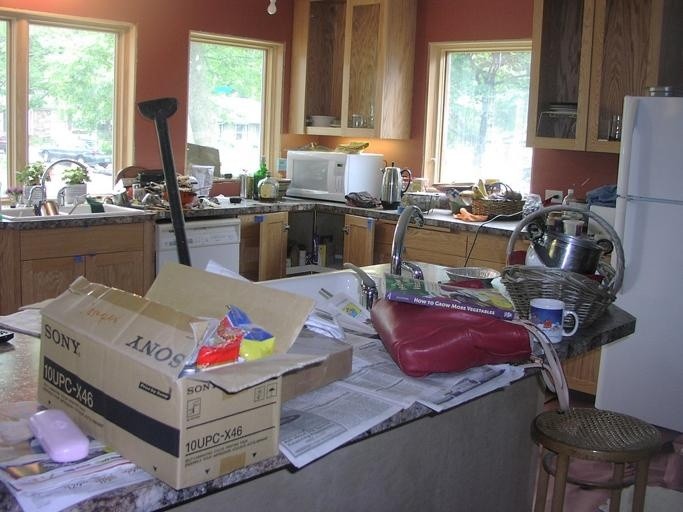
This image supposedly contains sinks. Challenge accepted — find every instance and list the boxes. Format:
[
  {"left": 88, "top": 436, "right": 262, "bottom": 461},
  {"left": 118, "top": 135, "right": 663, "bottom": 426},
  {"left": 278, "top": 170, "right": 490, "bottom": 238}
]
[
  {"left": 355, "top": 259, "right": 451, "bottom": 292},
  {"left": 0, "top": 206, "right": 68, "bottom": 221},
  {"left": 253, "top": 269, "right": 386, "bottom": 309},
  {"left": 55, "top": 203, "right": 144, "bottom": 216}
]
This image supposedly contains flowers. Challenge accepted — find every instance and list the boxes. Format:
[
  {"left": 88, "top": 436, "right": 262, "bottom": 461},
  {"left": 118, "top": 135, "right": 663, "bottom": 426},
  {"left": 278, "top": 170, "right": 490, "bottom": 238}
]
[{"left": 7, "top": 187, "right": 22, "bottom": 198}]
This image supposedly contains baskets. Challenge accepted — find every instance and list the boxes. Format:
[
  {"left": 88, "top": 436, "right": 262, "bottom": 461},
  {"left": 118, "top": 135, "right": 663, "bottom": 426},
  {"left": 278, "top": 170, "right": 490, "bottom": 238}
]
[
  {"left": 470, "top": 198, "right": 524, "bottom": 221},
  {"left": 502, "top": 202, "right": 625, "bottom": 330}
]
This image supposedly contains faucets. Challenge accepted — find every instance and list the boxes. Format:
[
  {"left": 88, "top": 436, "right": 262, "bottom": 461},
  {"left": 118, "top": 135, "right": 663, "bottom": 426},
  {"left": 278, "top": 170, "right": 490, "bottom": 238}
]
[
  {"left": 391, "top": 204, "right": 425, "bottom": 276},
  {"left": 38, "top": 158, "right": 89, "bottom": 206}
]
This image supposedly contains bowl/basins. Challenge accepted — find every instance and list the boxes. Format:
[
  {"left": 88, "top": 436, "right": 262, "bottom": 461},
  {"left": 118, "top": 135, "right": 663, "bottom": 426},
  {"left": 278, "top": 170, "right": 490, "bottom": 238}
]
[{"left": 645, "top": 84, "right": 682, "bottom": 96}]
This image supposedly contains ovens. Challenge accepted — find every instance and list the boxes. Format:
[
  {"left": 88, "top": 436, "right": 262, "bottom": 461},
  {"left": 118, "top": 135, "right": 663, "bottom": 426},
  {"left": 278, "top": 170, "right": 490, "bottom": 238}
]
[{"left": 156, "top": 215, "right": 240, "bottom": 282}]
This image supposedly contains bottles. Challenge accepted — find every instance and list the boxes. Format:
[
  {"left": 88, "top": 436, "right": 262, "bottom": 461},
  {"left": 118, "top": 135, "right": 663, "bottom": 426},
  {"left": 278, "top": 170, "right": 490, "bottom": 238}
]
[
  {"left": 298, "top": 246, "right": 304, "bottom": 266},
  {"left": 317, "top": 286, "right": 370, "bottom": 323},
  {"left": 562, "top": 187, "right": 577, "bottom": 218},
  {"left": 240, "top": 156, "right": 279, "bottom": 202},
  {"left": 130, "top": 183, "right": 141, "bottom": 199},
  {"left": 317, "top": 238, "right": 326, "bottom": 267}
]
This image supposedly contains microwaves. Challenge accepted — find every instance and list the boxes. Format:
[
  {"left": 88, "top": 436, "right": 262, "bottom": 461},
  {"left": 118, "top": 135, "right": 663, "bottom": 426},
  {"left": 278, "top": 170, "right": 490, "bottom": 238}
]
[{"left": 285, "top": 149, "right": 383, "bottom": 204}]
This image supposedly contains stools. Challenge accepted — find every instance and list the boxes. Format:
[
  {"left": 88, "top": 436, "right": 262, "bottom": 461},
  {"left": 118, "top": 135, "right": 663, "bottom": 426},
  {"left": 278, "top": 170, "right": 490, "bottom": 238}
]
[{"left": 531, "top": 407, "right": 661, "bottom": 512}]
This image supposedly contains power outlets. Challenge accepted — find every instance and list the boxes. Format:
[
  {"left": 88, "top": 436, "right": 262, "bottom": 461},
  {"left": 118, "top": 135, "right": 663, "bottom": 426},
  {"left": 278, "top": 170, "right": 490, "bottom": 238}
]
[{"left": 545, "top": 190, "right": 563, "bottom": 204}]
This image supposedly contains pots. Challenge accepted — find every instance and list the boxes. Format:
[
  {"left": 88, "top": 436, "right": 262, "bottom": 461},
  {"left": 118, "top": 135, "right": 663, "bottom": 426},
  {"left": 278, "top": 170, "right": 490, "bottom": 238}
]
[{"left": 524, "top": 220, "right": 613, "bottom": 276}]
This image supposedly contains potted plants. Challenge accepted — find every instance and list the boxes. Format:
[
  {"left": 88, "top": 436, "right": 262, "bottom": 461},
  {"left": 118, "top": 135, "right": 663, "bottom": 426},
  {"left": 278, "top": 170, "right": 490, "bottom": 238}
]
[
  {"left": 61, "top": 167, "right": 90, "bottom": 204},
  {"left": 16, "top": 161, "right": 50, "bottom": 202}
]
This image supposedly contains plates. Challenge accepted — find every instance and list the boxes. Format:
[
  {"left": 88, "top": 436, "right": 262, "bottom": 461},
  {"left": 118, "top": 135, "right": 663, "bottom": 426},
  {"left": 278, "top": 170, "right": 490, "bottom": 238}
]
[
  {"left": 305, "top": 115, "right": 340, "bottom": 127},
  {"left": 444, "top": 266, "right": 501, "bottom": 283}
]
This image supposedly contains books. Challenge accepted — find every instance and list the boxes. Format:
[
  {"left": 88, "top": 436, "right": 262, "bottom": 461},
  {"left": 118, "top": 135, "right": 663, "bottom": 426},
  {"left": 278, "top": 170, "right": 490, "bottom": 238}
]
[{"left": 379, "top": 272, "right": 514, "bottom": 322}]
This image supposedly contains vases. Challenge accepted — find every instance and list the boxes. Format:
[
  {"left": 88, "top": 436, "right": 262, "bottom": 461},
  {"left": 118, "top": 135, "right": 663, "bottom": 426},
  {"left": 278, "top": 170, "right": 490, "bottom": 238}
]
[{"left": 10, "top": 195, "right": 18, "bottom": 208}]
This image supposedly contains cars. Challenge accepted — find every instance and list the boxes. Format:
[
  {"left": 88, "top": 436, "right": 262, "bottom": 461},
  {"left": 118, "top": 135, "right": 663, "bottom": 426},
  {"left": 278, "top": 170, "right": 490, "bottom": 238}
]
[{"left": 0, "top": 127, "right": 112, "bottom": 171}]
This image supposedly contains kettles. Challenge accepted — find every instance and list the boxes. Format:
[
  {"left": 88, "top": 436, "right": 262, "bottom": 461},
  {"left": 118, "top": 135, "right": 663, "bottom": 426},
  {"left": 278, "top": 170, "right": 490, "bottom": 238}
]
[{"left": 378, "top": 162, "right": 410, "bottom": 209}]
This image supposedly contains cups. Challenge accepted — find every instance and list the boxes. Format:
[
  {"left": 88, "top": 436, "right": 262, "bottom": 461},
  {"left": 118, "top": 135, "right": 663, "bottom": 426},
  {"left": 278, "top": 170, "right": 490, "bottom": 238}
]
[
  {"left": 191, "top": 164, "right": 215, "bottom": 198},
  {"left": 562, "top": 219, "right": 583, "bottom": 236},
  {"left": 348, "top": 99, "right": 374, "bottom": 128},
  {"left": 527, "top": 297, "right": 578, "bottom": 345}
]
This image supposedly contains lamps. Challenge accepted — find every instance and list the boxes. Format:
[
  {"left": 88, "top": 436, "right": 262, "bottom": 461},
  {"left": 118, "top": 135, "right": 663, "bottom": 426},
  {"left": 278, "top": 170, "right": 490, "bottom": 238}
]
[{"left": 267, "top": 0, "right": 277, "bottom": 15}]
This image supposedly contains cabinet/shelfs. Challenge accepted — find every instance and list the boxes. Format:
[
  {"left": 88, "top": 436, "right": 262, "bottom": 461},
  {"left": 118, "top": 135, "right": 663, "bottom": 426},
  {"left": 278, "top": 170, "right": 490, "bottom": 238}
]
[
  {"left": 526, "top": 0, "right": 665, "bottom": 154},
  {"left": 1, "top": 222, "right": 156, "bottom": 317},
  {"left": 289, "top": 0, "right": 417, "bottom": 139}
]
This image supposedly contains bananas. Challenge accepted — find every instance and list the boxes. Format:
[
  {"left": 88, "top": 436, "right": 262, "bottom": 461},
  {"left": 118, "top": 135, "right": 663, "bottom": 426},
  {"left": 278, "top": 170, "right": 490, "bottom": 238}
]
[{"left": 459, "top": 179, "right": 488, "bottom": 199}]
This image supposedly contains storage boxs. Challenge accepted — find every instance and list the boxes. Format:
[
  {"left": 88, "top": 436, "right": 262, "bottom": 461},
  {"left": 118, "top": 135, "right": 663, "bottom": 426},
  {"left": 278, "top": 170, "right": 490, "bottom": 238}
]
[{"left": 38, "top": 260, "right": 327, "bottom": 491}]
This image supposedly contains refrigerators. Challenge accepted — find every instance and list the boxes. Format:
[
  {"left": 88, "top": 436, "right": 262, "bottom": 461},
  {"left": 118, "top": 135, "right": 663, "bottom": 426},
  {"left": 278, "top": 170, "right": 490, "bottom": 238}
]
[{"left": 591, "top": 94, "right": 682, "bottom": 434}]
